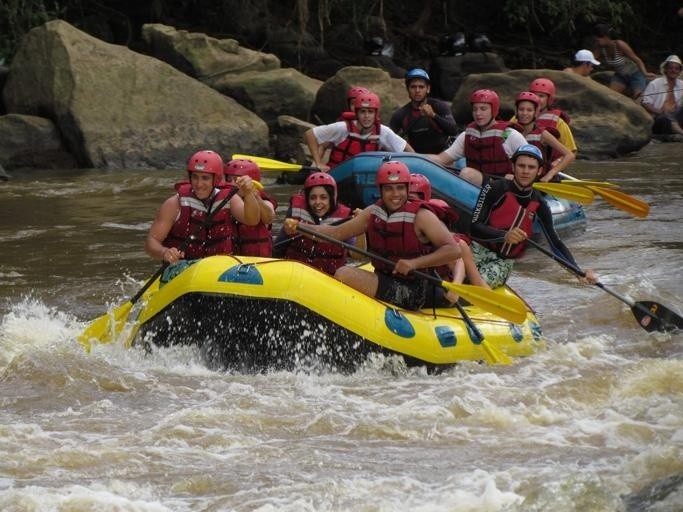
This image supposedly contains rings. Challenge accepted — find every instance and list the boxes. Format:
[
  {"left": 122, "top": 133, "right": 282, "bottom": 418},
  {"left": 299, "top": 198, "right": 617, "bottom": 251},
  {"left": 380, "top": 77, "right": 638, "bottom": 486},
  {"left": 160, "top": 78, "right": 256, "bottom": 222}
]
[
  {"left": 168, "top": 255, "right": 173, "bottom": 259},
  {"left": 174, "top": 251, "right": 179, "bottom": 257}
]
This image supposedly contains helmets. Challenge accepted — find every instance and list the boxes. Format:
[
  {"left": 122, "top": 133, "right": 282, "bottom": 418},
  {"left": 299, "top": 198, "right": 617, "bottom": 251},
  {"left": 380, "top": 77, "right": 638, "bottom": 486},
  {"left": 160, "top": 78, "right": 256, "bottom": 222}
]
[
  {"left": 469, "top": 89, "right": 500, "bottom": 118},
  {"left": 516, "top": 91, "right": 540, "bottom": 118},
  {"left": 303, "top": 172, "right": 338, "bottom": 205},
  {"left": 409, "top": 173, "right": 432, "bottom": 201},
  {"left": 530, "top": 78, "right": 556, "bottom": 106},
  {"left": 187, "top": 150, "right": 225, "bottom": 186},
  {"left": 376, "top": 160, "right": 409, "bottom": 193},
  {"left": 660, "top": 54, "right": 683, "bottom": 78},
  {"left": 511, "top": 144, "right": 543, "bottom": 164},
  {"left": 346, "top": 86, "right": 368, "bottom": 101},
  {"left": 354, "top": 92, "right": 380, "bottom": 121},
  {"left": 404, "top": 67, "right": 432, "bottom": 85},
  {"left": 223, "top": 159, "right": 261, "bottom": 184}
]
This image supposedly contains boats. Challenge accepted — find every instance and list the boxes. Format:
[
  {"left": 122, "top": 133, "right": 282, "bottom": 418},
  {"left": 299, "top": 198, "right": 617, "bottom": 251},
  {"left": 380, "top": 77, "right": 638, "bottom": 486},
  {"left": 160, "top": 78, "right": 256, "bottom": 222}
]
[
  {"left": 125, "top": 253, "right": 544, "bottom": 379},
  {"left": 323, "top": 151, "right": 590, "bottom": 244}
]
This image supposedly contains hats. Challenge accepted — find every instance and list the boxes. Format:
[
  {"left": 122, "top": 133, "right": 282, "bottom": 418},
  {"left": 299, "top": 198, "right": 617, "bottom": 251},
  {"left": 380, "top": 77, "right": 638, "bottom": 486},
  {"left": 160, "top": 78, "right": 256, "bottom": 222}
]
[{"left": 576, "top": 49, "right": 602, "bottom": 67}]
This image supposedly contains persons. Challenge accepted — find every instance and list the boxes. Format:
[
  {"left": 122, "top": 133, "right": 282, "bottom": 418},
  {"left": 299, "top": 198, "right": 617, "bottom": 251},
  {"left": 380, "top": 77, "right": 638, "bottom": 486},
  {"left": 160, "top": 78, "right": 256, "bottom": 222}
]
[
  {"left": 389, "top": 67, "right": 459, "bottom": 155},
  {"left": 421, "top": 86, "right": 530, "bottom": 189},
  {"left": 641, "top": 54, "right": 683, "bottom": 135},
  {"left": 461, "top": 143, "right": 598, "bottom": 290},
  {"left": 271, "top": 171, "right": 367, "bottom": 282},
  {"left": 222, "top": 159, "right": 279, "bottom": 259},
  {"left": 303, "top": 90, "right": 417, "bottom": 175},
  {"left": 408, "top": 172, "right": 470, "bottom": 315},
  {"left": 466, "top": 28, "right": 491, "bottom": 53},
  {"left": 586, "top": 24, "right": 657, "bottom": 99},
  {"left": 311, "top": 85, "right": 369, "bottom": 168},
  {"left": 144, "top": 147, "right": 262, "bottom": 292},
  {"left": 561, "top": 48, "right": 601, "bottom": 80},
  {"left": 509, "top": 77, "right": 578, "bottom": 171},
  {"left": 361, "top": 26, "right": 399, "bottom": 57},
  {"left": 281, "top": 159, "right": 461, "bottom": 309},
  {"left": 510, "top": 90, "right": 577, "bottom": 197},
  {"left": 436, "top": 24, "right": 466, "bottom": 57}
]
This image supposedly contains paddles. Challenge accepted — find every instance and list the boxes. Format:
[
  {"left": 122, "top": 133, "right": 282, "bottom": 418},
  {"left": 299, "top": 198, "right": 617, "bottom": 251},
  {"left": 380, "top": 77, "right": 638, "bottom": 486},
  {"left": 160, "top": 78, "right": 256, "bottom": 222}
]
[
  {"left": 284, "top": 219, "right": 527, "bottom": 369},
  {"left": 78, "top": 179, "right": 263, "bottom": 354},
  {"left": 232, "top": 153, "right": 322, "bottom": 172},
  {"left": 444, "top": 167, "right": 650, "bottom": 218},
  {"left": 526, "top": 236, "right": 682, "bottom": 333}
]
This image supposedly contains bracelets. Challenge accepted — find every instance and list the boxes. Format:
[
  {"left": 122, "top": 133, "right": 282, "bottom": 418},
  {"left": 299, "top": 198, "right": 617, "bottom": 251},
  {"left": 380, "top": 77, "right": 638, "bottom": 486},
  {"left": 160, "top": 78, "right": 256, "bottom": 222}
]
[{"left": 557, "top": 158, "right": 562, "bottom": 162}]
